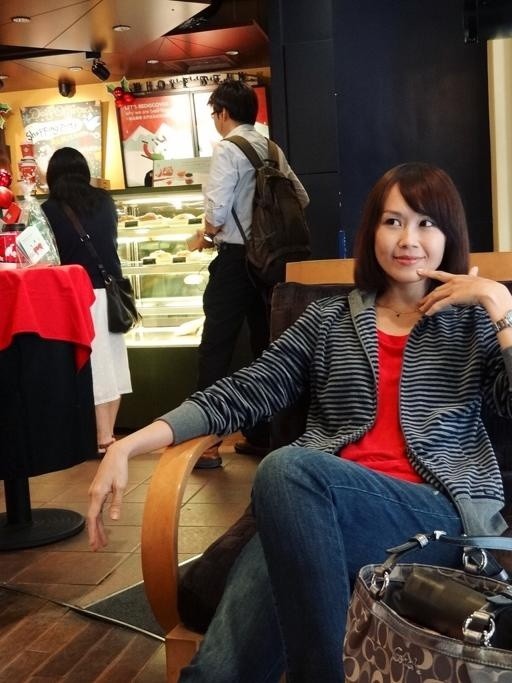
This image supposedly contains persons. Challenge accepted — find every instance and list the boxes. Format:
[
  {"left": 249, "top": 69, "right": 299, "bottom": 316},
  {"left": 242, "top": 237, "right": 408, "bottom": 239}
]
[
  {"left": 33, "top": 145, "right": 137, "bottom": 455},
  {"left": 80, "top": 157, "right": 511, "bottom": 683},
  {"left": 174, "top": 78, "right": 313, "bottom": 471}
]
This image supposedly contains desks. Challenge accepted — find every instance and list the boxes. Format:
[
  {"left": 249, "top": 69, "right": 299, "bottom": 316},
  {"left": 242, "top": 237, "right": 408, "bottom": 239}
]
[{"left": 1, "top": 262, "right": 101, "bottom": 553}]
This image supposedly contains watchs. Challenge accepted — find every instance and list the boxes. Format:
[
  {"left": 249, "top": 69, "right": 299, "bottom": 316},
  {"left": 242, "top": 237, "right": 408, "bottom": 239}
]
[{"left": 491, "top": 308, "right": 511, "bottom": 335}]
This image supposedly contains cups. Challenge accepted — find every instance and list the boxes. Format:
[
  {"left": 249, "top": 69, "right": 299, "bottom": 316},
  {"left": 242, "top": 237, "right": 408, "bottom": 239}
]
[{"left": 19, "top": 142, "right": 33, "bottom": 159}]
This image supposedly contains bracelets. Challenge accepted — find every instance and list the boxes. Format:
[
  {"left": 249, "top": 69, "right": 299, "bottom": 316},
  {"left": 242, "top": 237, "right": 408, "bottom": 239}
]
[{"left": 200, "top": 227, "right": 215, "bottom": 243}]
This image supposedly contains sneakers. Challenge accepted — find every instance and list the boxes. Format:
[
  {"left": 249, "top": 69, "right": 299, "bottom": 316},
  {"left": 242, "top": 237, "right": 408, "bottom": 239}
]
[
  {"left": 235, "top": 439, "right": 271, "bottom": 456},
  {"left": 197, "top": 447, "right": 222, "bottom": 468}
]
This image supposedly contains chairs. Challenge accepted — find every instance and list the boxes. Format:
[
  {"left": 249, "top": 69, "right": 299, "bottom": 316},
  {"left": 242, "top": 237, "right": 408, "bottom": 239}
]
[{"left": 141, "top": 248, "right": 511, "bottom": 683}]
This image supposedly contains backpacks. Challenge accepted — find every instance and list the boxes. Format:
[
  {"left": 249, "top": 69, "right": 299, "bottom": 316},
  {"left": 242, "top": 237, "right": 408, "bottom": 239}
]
[{"left": 219, "top": 135, "right": 313, "bottom": 281}]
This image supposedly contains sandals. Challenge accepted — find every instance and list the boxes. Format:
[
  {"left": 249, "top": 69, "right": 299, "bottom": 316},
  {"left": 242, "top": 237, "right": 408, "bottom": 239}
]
[{"left": 97, "top": 434, "right": 116, "bottom": 459}]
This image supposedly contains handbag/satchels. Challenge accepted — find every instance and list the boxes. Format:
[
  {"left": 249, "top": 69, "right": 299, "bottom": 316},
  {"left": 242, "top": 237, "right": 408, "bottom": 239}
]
[
  {"left": 105, "top": 277, "right": 141, "bottom": 333},
  {"left": 342, "top": 531, "right": 512, "bottom": 683}
]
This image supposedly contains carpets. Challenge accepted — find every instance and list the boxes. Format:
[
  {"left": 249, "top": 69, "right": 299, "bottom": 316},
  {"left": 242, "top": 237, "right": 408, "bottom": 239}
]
[
  {"left": 0, "top": 582, "right": 168, "bottom": 682},
  {"left": 82, "top": 551, "right": 208, "bottom": 641}
]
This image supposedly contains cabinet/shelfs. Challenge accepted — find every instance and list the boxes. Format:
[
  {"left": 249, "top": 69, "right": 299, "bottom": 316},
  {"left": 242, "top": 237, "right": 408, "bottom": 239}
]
[{"left": 9, "top": 185, "right": 222, "bottom": 435}]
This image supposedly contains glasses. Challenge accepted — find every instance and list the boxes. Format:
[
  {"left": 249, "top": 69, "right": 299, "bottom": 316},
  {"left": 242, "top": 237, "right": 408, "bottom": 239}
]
[{"left": 211, "top": 111, "right": 217, "bottom": 118}]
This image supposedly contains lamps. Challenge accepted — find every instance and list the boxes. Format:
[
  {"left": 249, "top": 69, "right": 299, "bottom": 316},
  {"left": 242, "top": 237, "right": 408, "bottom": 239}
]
[{"left": 86, "top": 50, "right": 111, "bottom": 81}]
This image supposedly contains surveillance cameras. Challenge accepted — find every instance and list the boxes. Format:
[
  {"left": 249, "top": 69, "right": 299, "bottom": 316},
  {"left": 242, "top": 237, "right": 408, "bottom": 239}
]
[{"left": 59, "top": 80, "right": 71, "bottom": 95}]
